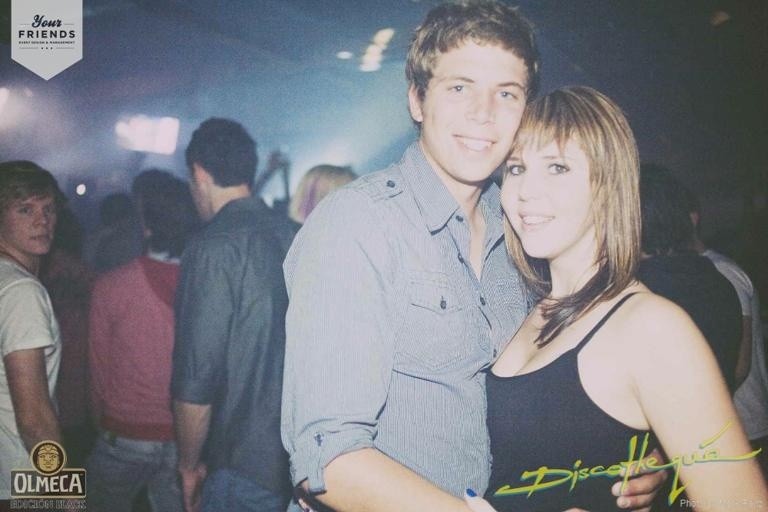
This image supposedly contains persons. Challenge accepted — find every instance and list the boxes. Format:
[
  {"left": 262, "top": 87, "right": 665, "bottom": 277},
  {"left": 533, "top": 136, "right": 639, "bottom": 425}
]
[
  {"left": 67, "top": 169, "right": 167, "bottom": 271},
  {"left": 281, "top": 1, "right": 669, "bottom": 512},
  {"left": 84, "top": 177, "right": 201, "bottom": 511},
  {"left": 43, "top": 184, "right": 90, "bottom": 452},
  {"left": 289, "top": 165, "right": 360, "bottom": 223},
  {"left": 248, "top": 147, "right": 295, "bottom": 216},
  {"left": 172, "top": 119, "right": 304, "bottom": 512},
  {"left": 684, "top": 186, "right": 768, "bottom": 476},
  {"left": 483, "top": 86, "right": 768, "bottom": 511},
  {"left": 632, "top": 164, "right": 739, "bottom": 404},
  {"left": 1, "top": 160, "right": 70, "bottom": 512}
]
